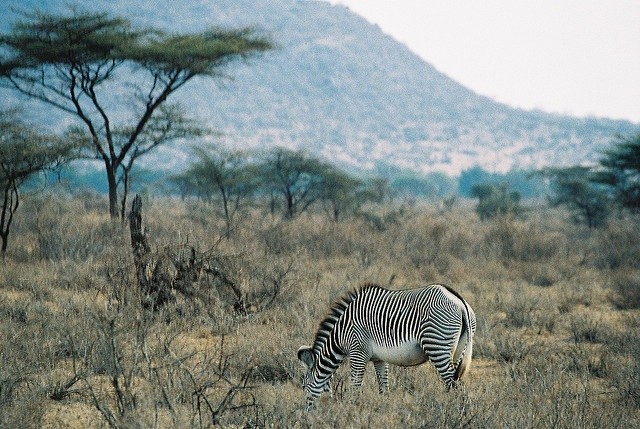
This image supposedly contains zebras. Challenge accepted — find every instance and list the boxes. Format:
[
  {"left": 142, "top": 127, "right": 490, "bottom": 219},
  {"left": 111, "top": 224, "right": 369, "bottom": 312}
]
[{"left": 298, "top": 283, "right": 477, "bottom": 412}]
